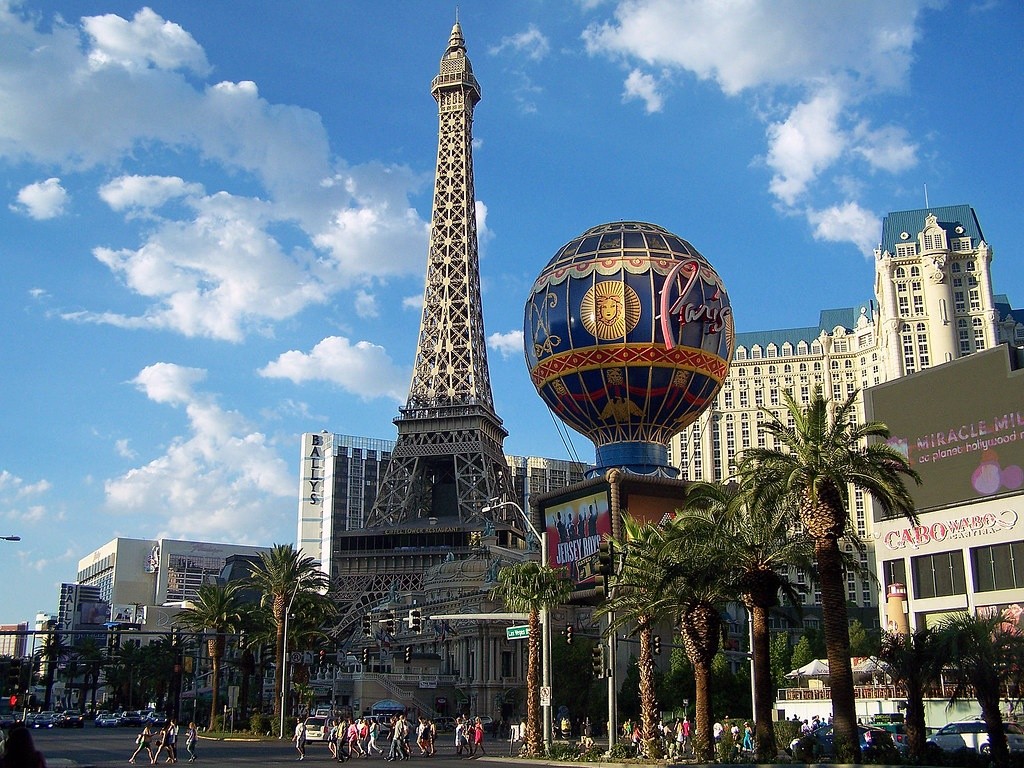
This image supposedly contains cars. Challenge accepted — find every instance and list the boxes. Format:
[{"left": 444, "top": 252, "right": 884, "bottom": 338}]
[
  {"left": 302, "top": 714, "right": 349, "bottom": 746},
  {"left": 790, "top": 720, "right": 896, "bottom": 764},
  {"left": 314, "top": 707, "right": 494, "bottom": 735},
  {"left": 874, "top": 722, "right": 909, "bottom": 755},
  {"left": 924, "top": 720, "right": 1024, "bottom": 758},
  {"left": 0, "top": 695, "right": 168, "bottom": 730}
]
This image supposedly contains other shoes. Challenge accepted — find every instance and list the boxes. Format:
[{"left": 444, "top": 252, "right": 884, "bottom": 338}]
[{"left": 129, "top": 760, "right": 134, "bottom": 764}]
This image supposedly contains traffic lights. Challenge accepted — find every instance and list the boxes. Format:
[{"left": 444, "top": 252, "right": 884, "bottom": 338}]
[
  {"left": 565, "top": 624, "right": 573, "bottom": 645},
  {"left": 318, "top": 649, "right": 326, "bottom": 667},
  {"left": 594, "top": 564, "right": 608, "bottom": 598},
  {"left": 362, "top": 612, "right": 373, "bottom": 637},
  {"left": 386, "top": 610, "right": 396, "bottom": 636},
  {"left": 410, "top": 607, "right": 422, "bottom": 636},
  {"left": 599, "top": 541, "right": 615, "bottom": 577},
  {"left": 652, "top": 634, "right": 662, "bottom": 655},
  {"left": 404, "top": 644, "right": 413, "bottom": 665},
  {"left": 591, "top": 643, "right": 606, "bottom": 679},
  {"left": 361, "top": 646, "right": 370, "bottom": 664},
  {"left": 10, "top": 692, "right": 20, "bottom": 706}
]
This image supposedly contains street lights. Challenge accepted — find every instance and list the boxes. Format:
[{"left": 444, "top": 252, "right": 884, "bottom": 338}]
[
  {"left": 280, "top": 569, "right": 316, "bottom": 739},
  {"left": 482, "top": 495, "right": 552, "bottom": 759}
]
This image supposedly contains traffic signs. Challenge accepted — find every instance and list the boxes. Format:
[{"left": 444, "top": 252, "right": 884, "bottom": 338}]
[
  {"left": 303, "top": 650, "right": 314, "bottom": 664},
  {"left": 336, "top": 648, "right": 348, "bottom": 662},
  {"left": 380, "top": 647, "right": 390, "bottom": 661}
]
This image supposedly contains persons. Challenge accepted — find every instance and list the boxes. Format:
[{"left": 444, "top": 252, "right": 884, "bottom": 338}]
[
  {"left": 129, "top": 722, "right": 157, "bottom": 765},
  {"left": 743, "top": 722, "right": 753, "bottom": 750},
  {"left": 713, "top": 719, "right": 724, "bottom": 750},
  {"left": 554, "top": 496, "right": 598, "bottom": 544},
  {"left": 623, "top": 719, "right": 643, "bottom": 752},
  {"left": 455, "top": 713, "right": 486, "bottom": 757},
  {"left": 552, "top": 718, "right": 571, "bottom": 739},
  {"left": 328, "top": 717, "right": 384, "bottom": 762},
  {"left": 154, "top": 719, "right": 179, "bottom": 764},
  {"left": 382, "top": 713, "right": 414, "bottom": 761},
  {"left": 658, "top": 715, "right": 697, "bottom": 757},
  {"left": 292, "top": 716, "right": 306, "bottom": 760},
  {"left": 730, "top": 722, "right": 740, "bottom": 740},
  {"left": 519, "top": 719, "right": 528, "bottom": 751},
  {"left": 579, "top": 721, "right": 594, "bottom": 748},
  {"left": 858, "top": 718, "right": 863, "bottom": 726},
  {"left": 492, "top": 718, "right": 509, "bottom": 738},
  {"left": 416, "top": 716, "right": 437, "bottom": 757},
  {"left": 0, "top": 728, "right": 48, "bottom": 768},
  {"left": 185, "top": 722, "right": 197, "bottom": 762},
  {"left": 787, "top": 713, "right": 833, "bottom": 732}
]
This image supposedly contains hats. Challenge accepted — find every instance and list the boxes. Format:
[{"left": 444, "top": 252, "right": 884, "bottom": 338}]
[{"left": 743, "top": 722, "right": 750, "bottom": 726}]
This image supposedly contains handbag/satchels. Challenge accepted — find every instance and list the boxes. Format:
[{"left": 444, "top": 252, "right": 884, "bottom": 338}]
[{"left": 136, "top": 734, "right": 145, "bottom": 744}]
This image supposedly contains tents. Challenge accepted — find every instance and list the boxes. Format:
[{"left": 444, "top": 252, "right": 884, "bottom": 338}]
[
  {"left": 784, "top": 659, "right": 829, "bottom": 687},
  {"left": 854, "top": 655, "right": 889, "bottom": 684}
]
